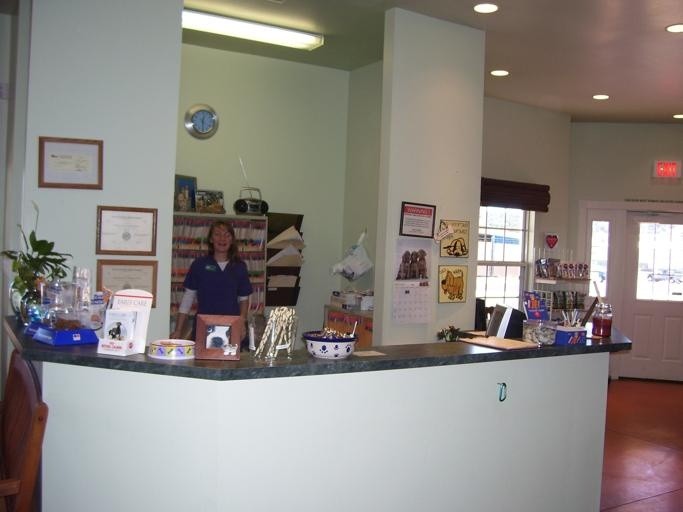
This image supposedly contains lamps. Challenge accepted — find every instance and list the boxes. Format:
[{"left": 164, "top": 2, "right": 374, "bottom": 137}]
[{"left": 180, "top": 8, "right": 323, "bottom": 52}]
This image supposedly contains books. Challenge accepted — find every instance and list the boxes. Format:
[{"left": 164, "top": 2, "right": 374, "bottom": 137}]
[
  {"left": 170, "top": 218, "right": 211, "bottom": 340},
  {"left": 231, "top": 220, "right": 262, "bottom": 317},
  {"left": 460, "top": 335, "right": 539, "bottom": 349}
]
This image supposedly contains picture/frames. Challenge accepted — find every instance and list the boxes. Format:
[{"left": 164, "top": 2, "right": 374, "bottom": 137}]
[
  {"left": 439, "top": 219, "right": 470, "bottom": 259},
  {"left": 398, "top": 200, "right": 436, "bottom": 239},
  {"left": 96, "top": 259, "right": 158, "bottom": 307},
  {"left": 38, "top": 135, "right": 103, "bottom": 189},
  {"left": 436, "top": 264, "right": 468, "bottom": 304},
  {"left": 95, "top": 204, "right": 158, "bottom": 256}
]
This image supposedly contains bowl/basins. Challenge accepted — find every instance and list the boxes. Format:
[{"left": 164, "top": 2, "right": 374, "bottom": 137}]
[
  {"left": 523, "top": 319, "right": 557, "bottom": 346},
  {"left": 302, "top": 331, "right": 358, "bottom": 360},
  {"left": 148, "top": 339, "right": 195, "bottom": 360}
]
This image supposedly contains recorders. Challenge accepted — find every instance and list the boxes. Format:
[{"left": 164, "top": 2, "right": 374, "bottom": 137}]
[{"left": 233, "top": 188, "right": 268, "bottom": 215}]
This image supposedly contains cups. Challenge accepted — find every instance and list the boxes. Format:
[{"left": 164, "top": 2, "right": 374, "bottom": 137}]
[{"left": 72, "top": 267, "right": 91, "bottom": 301}]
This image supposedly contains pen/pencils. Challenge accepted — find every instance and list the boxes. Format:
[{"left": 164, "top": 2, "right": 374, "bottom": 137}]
[{"left": 559, "top": 308, "right": 581, "bottom": 328}]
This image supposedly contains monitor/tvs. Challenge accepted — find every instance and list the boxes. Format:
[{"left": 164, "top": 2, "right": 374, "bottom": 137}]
[{"left": 485, "top": 304, "right": 527, "bottom": 338}]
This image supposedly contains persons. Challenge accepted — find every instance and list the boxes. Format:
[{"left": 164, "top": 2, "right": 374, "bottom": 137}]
[{"left": 170, "top": 221, "right": 251, "bottom": 349}]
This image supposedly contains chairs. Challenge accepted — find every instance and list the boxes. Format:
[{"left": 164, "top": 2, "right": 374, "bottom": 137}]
[{"left": 0, "top": 349, "right": 47, "bottom": 512}]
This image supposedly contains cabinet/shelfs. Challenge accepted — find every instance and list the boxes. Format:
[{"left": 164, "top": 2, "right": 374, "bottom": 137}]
[
  {"left": 172, "top": 211, "right": 268, "bottom": 334},
  {"left": 532, "top": 245, "right": 590, "bottom": 321}
]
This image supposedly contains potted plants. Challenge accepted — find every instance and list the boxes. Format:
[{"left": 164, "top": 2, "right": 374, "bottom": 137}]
[
  {"left": 0, "top": 201, "right": 44, "bottom": 317},
  {"left": 13, "top": 231, "right": 73, "bottom": 324}
]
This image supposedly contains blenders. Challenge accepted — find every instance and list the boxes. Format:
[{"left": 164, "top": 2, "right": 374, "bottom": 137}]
[{"left": 23, "top": 276, "right": 99, "bottom": 346}]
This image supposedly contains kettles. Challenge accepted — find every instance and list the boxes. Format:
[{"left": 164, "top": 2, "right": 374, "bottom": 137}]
[{"left": 19, "top": 290, "right": 39, "bottom": 325}]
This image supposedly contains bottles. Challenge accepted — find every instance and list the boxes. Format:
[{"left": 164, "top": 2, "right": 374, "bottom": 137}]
[{"left": 592, "top": 303, "right": 613, "bottom": 338}]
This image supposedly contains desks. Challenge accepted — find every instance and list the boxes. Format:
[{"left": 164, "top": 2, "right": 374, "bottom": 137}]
[{"left": 323, "top": 303, "right": 372, "bottom": 348}]
